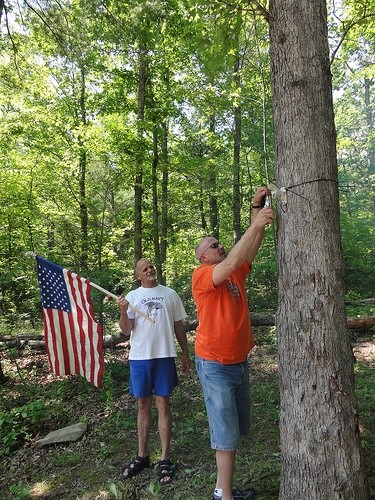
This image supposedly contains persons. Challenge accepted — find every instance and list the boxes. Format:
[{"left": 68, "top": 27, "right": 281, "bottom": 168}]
[
  {"left": 118, "top": 259, "right": 191, "bottom": 486},
  {"left": 191, "top": 184, "right": 276, "bottom": 500}
]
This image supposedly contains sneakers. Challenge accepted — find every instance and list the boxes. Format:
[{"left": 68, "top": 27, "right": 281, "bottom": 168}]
[{"left": 211, "top": 488, "right": 255, "bottom": 500}]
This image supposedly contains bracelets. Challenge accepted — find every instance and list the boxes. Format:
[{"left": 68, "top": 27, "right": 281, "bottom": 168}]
[{"left": 252, "top": 204, "right": 262, "bottom": 209}]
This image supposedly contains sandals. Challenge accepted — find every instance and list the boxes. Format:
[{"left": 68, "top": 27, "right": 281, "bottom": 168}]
[
  {"left": 158, "top": 458, "right": 174, "bottom": 484},
  {"left": 121, "top": 454, "right": 151, "bottom": 479}
]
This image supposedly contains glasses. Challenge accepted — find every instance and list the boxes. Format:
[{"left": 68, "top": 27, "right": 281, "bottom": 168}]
[{"left": 201, "top": 241, "right": 221, "bottom": 256}]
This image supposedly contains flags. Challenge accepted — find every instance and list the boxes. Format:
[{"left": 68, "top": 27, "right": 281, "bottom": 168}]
[{"left": 37, "top": 255, "right": 105, "bottom": 388}]
